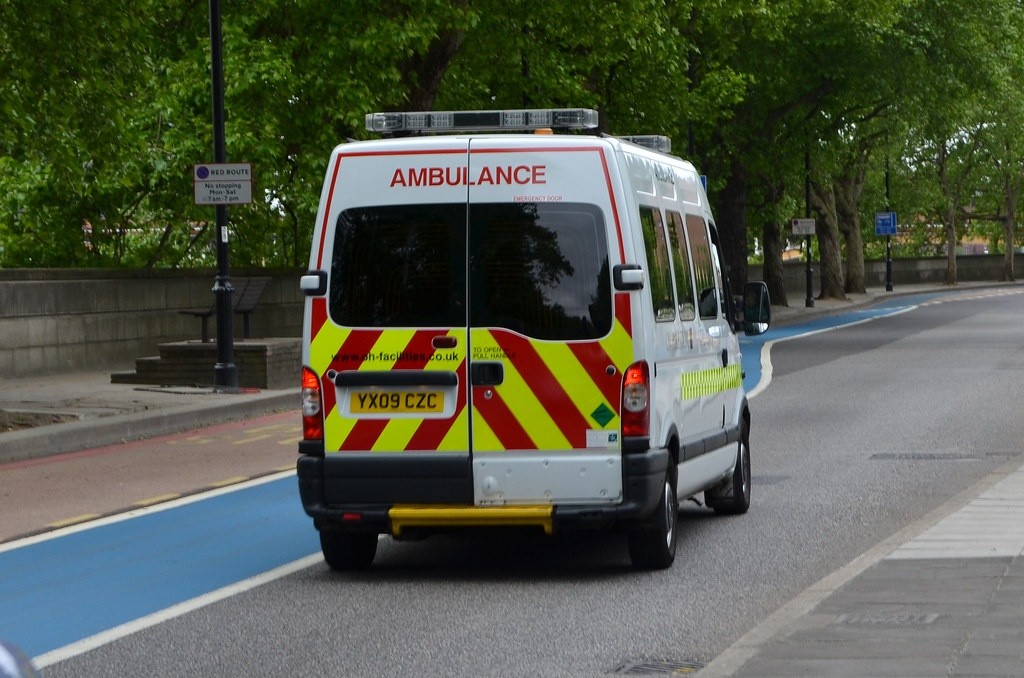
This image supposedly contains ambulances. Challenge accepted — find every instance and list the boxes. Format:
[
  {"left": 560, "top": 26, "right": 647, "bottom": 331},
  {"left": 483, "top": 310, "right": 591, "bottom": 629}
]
[{"left": 294, "top": 103, "right": 774, "bottom": 570}]
